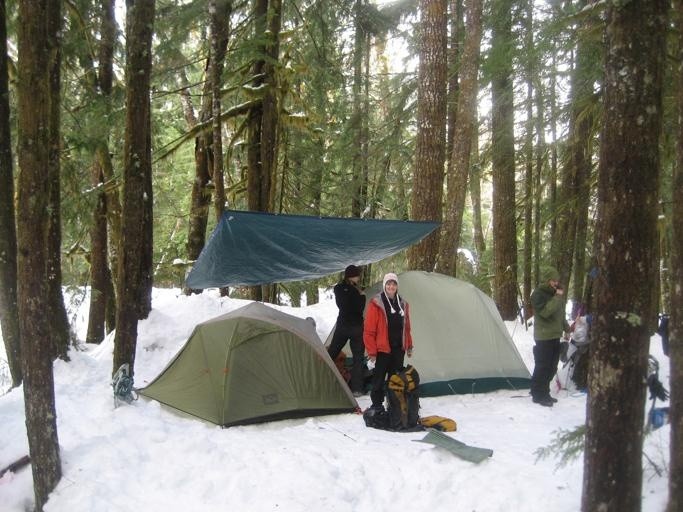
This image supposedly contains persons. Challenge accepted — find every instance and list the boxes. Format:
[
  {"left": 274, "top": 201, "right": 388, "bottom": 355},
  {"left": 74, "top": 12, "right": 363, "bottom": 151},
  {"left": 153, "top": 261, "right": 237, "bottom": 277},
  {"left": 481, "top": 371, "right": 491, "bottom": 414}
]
[
  {"left": 329, "top": 265, "right": 366, "bottom": 396},
  {"left": 530, "top": 265, "right": 569, "bottom": 407},
  {"left": 362, "top": 272, "right": 412, "bottom": 409}
]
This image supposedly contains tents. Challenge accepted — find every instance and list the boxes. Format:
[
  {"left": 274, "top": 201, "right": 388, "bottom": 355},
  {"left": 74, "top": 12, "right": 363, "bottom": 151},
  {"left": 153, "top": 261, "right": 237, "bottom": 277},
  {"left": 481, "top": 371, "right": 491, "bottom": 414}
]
[
  {"left": 322, "top": 270, "right": 532, "bottom": 397},
  {"left": 136, "top": 302, "right": 361, "bottom": 428}
]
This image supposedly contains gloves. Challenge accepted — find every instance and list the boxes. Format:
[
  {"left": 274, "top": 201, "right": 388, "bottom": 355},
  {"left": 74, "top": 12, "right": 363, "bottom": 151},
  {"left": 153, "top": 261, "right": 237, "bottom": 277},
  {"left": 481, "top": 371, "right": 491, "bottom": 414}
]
[{"left": 367, "top": 359, "right": 376, "bottom": 370}]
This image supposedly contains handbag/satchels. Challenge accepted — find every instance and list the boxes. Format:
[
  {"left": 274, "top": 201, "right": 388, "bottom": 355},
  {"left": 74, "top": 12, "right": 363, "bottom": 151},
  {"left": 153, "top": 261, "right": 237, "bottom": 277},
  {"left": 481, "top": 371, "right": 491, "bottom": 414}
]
[
  {"left": 382, "top": 364, "right": 421, "bottom": 430},
  {"left": 348, "top": 368, "right": 374, "bottom": 395}
]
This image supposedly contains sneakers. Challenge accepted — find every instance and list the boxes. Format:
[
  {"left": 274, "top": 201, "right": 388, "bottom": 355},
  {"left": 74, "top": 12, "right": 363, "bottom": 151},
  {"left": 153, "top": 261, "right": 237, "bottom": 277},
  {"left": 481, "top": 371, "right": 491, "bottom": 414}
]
[{"left": 533, "top": 396, "right": 557, "bottom": 406}]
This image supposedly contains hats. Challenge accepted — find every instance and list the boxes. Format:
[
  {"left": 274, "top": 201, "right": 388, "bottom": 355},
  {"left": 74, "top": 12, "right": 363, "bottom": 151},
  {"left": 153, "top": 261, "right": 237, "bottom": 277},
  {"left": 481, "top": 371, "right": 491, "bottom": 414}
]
[
  {"left": 382, "top": 273, "right": 405, "bottom": 317},
  {"left": 345, "top": 265, "right": 360, "bottom": 277}
]
